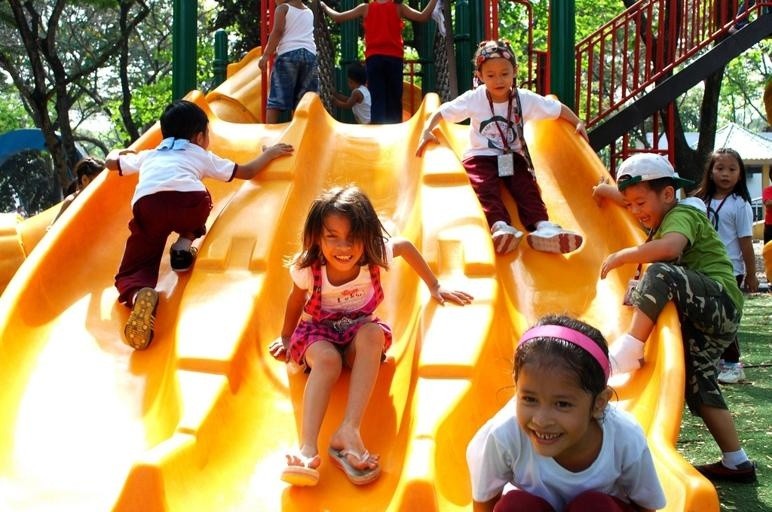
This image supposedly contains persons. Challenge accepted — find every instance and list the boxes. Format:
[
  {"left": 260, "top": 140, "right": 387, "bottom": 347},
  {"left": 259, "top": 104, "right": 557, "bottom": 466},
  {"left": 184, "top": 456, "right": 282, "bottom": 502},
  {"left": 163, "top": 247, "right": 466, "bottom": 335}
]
[
  {"left": 687, "top": 148, "right": 759, "bottom": 384},
  {"left": 268, "top": 186, "right": 474, "bottom": 485},
  {"left": 415, "top": 41, "right": 590, "bottom": 254},
  {"left": 105, "top": 100, "right": 295, "bottom": 350},
  {"left": 258, "top": 0, "right": 317, "bottom": 123},
  {"left": 762, "top": 164, "right": 772, "bottom": 244},
  {"left": 593, "top": 152, "right": 757, "bottom": 483},
  {"left": 328, "top": 64, "right": 372, "bottom": 124},
  {"left": 466, "top": 315, "right": 667, "bottom": 512},
  {"left": 319, "top": 0, "right": 438, "bottom": 124},
  {"left": 51, "top": 158, "right": 105, "bottom": 225}
]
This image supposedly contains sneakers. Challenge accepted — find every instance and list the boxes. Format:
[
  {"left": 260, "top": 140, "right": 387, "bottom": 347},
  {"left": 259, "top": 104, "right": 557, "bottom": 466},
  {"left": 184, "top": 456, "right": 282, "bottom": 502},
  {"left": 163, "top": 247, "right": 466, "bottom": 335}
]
[
  {"left": 692, "top": 459, "right": 758, "bottom": 484},
  {"left": 526, "top": 223, "right": 582, "bottom": 255},
  {"left": 491, "top": 225, "right": 524, "bottom": 255},
  {"left": 716, "top": 364, "right": 745, "bottom": 384},
  {"left": 170, "top": 242, "right": 199, "bottom": 271},
  {"left": 123, "top": 287, "right": 160, "bottom": 350}
]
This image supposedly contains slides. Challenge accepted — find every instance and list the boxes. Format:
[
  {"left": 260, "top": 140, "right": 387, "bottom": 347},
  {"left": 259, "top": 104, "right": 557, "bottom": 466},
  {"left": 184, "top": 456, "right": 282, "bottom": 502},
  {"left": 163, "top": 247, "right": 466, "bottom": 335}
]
[{"left": 0, "top": 90, "right": 721, "bottom": 511}]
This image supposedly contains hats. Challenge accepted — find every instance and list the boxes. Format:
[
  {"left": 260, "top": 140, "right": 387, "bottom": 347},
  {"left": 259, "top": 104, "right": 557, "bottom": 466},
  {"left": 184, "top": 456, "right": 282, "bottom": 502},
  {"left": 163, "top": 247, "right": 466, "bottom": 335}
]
[{"left": 617, "top": 153, "right": 694, "bottom": 191}]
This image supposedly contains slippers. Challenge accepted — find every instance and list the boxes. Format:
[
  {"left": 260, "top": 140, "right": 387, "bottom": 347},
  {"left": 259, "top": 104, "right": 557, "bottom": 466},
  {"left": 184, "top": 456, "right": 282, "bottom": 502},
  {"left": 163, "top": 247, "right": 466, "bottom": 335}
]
[
  {"left": 328, "top": 444, "right": 382, "bottom": 486},
  {"left": 282, "top": 452, "right": 319, "bottom": 488}
]
